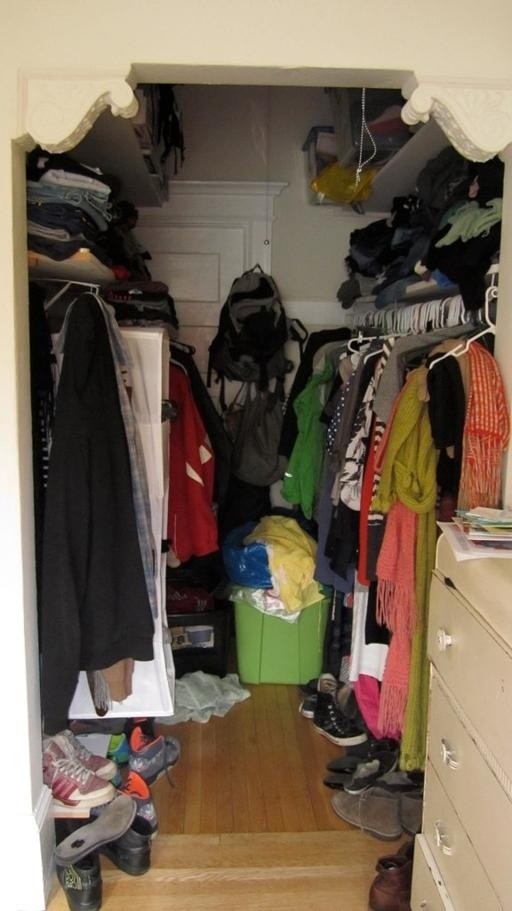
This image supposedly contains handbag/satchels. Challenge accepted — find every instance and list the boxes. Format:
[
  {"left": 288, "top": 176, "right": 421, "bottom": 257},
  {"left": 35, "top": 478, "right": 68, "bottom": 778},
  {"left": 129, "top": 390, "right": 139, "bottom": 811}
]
[{"left": 222, "top": 368, "right": 286, "bottom": 488}]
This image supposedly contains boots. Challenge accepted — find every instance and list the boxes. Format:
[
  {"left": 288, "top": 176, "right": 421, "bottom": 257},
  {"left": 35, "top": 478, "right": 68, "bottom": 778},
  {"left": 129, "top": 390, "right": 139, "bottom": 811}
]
[
  {"left": 368, "top": 840, "right": 416, "bottom": 911},
  {"left": 331, "top": 792, "right": 401, "bottom": 840},
  {"left": 368, "top": 782, "right": 425, "bottom": 835}
]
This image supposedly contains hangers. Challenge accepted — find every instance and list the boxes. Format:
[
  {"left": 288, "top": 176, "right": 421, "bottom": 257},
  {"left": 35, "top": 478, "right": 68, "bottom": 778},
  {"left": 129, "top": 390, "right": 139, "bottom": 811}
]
[{"left": 345, "top": 284, "right": 499, "bottom": 374}]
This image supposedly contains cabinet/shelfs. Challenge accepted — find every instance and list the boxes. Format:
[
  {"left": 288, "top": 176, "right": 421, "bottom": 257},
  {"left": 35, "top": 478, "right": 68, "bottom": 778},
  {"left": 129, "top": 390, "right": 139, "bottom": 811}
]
[
  {"left": 409, "top": 532, "right": 512, "bottom": 911},
  {"left": 353, "top": 117, "right": 500, "bottom": 305}
]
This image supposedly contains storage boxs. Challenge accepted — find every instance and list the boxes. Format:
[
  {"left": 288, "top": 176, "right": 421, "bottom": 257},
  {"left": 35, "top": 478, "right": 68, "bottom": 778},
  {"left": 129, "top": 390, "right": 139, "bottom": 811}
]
[
  {"left": 300, "top": 88, "right": 416, "bottom": 208},
  {"left": 231, "top": 582, "right": 332, "bottom": 688}
]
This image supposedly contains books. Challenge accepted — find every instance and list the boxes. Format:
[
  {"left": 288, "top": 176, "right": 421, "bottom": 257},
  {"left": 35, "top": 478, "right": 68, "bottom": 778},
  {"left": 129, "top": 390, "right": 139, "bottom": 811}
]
[{"left": 433, "top": 505, "right": 512, "bottom": 562}]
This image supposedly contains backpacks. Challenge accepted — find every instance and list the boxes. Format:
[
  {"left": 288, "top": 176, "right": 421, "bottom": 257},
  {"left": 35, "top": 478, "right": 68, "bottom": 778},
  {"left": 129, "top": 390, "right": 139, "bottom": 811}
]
[{"left": 204, "top": 270, "right": 286, "bottom": 381}]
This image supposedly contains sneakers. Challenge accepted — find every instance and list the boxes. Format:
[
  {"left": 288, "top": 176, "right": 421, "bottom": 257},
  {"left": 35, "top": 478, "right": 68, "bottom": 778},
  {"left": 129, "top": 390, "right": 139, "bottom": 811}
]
[
  {"left": 299, "top": 673, "right": 424, "bottom": 795},
  {"left": 42, "top": 718, "right": 181, "bottom": 911}
]
